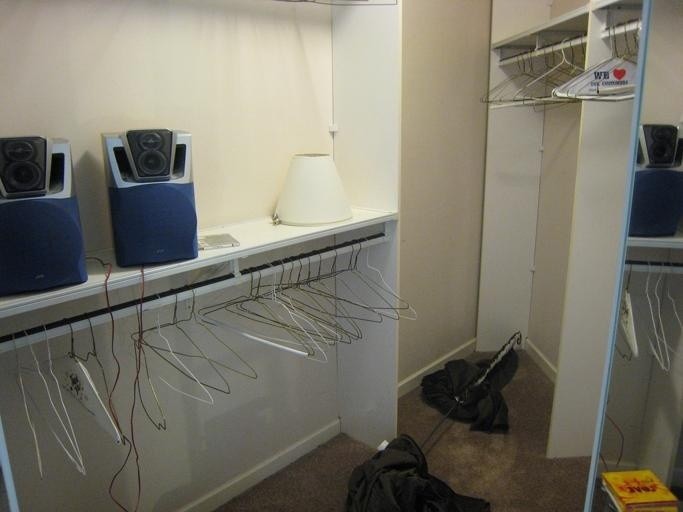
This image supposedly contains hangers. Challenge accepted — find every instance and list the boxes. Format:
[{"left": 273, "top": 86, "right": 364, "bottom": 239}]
[
  {"left": 476, "top": 18, "right": 644, "bottom": 113},
  {"left": 613, "top": 256, "right": 682, "bottom": 374}
]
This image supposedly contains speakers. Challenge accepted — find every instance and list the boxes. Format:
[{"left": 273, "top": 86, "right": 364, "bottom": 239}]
[
  {"left": 0, "top": 136, "right": 88, "bottom": 296},
  {"left": 101, "top": 128, "right": 198, "bottom": 268},
  {"left": 628, "top": 124, "right": 683, "bottom": 238}
]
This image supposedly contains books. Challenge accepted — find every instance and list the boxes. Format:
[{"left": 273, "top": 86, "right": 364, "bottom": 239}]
[{"left": 598, "top": 466, "right": 680, "bottom": 510}]
